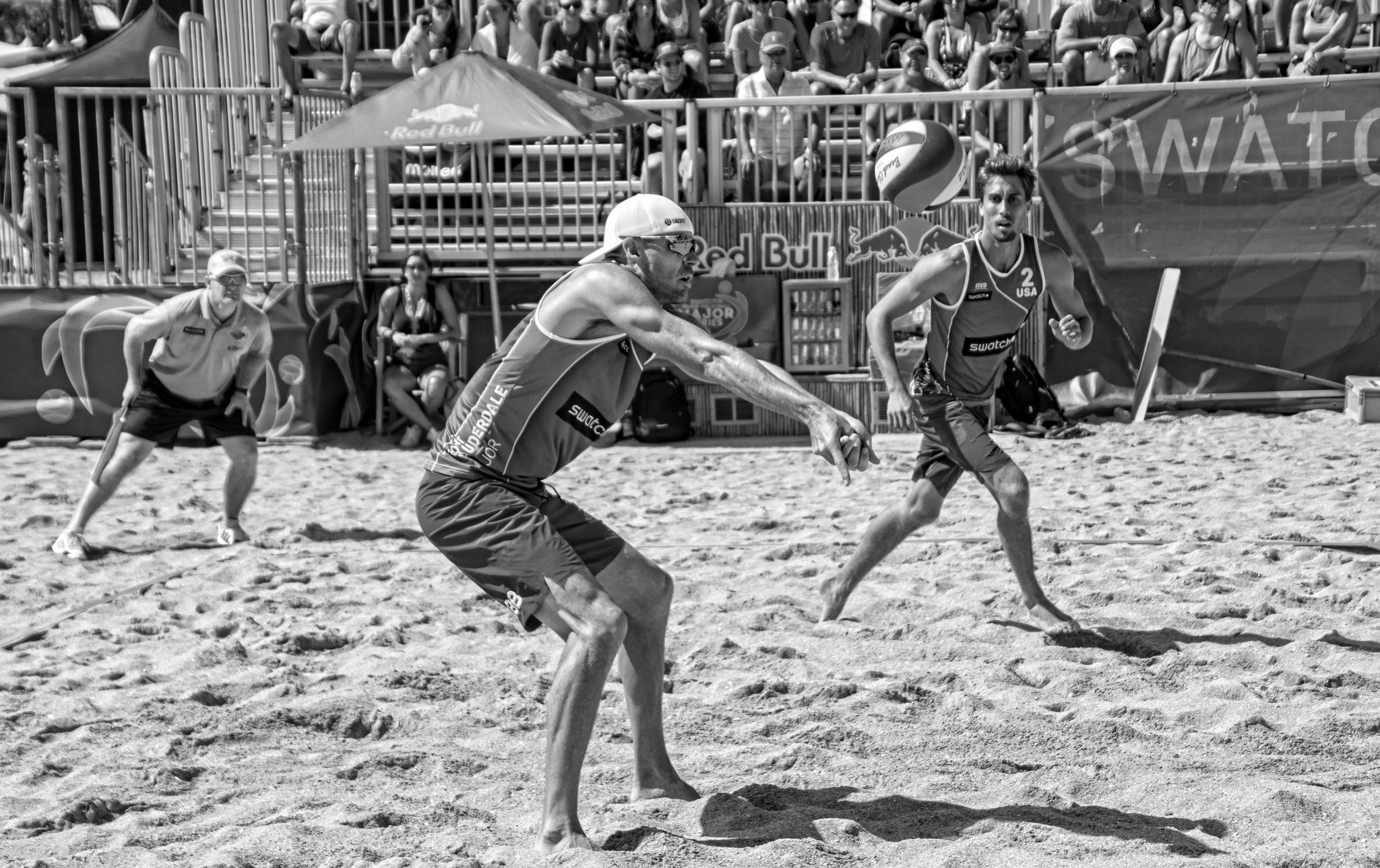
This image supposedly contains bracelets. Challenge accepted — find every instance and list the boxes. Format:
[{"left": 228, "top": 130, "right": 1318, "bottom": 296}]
[{"left": 390, "top": 330, "right": 399, "bottom": 341}]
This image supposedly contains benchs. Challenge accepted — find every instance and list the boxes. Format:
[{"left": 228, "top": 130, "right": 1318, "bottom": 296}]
[
  {"left": 643, "top": 275, "right": 779, "bottom": 436},
  {"left": 290, "top": 13, "right": 1380, "bottom": 190}
]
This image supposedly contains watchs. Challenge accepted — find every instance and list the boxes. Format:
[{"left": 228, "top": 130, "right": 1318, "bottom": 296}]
[{"left": 236, "top": 387, "right": 250, "bottom": 397}]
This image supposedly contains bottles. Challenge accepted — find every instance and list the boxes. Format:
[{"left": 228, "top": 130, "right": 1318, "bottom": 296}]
[
  {"left": 840, "top": 155, "right": 850, "bottom": 178},
  {"left": 1114, "top": 407, "right": 1132, "bottom": 422},
  {"left": 827, "top": 247, "right": 839, "bottom": 280}
]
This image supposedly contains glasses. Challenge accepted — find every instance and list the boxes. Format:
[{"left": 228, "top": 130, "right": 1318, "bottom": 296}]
[
  {"left": 405, "top": 262, "right": 426, "bottom": 271},
  {"left": 993, "top": 55, "right": 1013, "bottom": 64},
  {"left": 434, "top": 5, "right": 450, "bottom": 10},
  {"left": 208, "top": 272, "right": 246, "bottom": 285},
  {"left": 660, "top": 58, "right": 681, "bottom": 67},
  {"left": 1207, "top": 0, "right": 1229, "bottom": 4},
  {"left": 945, "top": 0, "right": 963, "bottom": 5},
  {"left": 1115, "top": 52, "right": 1134, "bottom": 58},
  {"left": 558, "top": 1, "right": 581, "bottom": 10},
  {"left": 998, "top": 23, "right": 1019, "bottom": 33},
  {"left": 766, "top": 50, "right": 785, "bottom": 56},
  {"left": 832, "top": 8, "right": 858, "bottom": 20},
  {"left": 750, "top": 0, "right": 769, "bottom": 5},
  {"left": 617, "top": 230, "right": 696, "bottom": 256}
]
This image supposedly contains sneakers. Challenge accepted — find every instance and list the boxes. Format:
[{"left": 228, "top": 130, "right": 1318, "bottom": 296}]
[
  {"left": 51, "top": 527, "right": 88, "bottom": 561},
  {"left": 217, "top": 521, "right": 250, "bottom": 546}
]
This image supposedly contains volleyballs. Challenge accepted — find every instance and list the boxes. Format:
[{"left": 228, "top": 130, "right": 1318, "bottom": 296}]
[{"left": 873, "top": 120, "right": 968, "bottom": 216}]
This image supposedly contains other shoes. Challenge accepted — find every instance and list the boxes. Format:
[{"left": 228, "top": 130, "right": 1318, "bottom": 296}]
[
  {"left": 401, "top": 426, "right": 421, "bottom": 447},
  {"left": 426, "top": 431, "right": 442, "bottom": 445}
]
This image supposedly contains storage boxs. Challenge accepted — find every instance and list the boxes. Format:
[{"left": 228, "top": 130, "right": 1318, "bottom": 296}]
[{"left": 1345, "top": 376, "right": 1380, "bottom": 421}]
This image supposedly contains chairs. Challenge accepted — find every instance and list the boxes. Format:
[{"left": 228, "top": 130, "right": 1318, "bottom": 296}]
[
  {"left": 867, "top": 272, "right": 995, "bottom": 434},
  {"left": 375, "top": 313, "right": 467, "bottom": 435}
]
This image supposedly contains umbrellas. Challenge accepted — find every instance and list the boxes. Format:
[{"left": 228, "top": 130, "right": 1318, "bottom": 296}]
[{"left": 275, "top": 47, "right": 673, "bottom": 351}]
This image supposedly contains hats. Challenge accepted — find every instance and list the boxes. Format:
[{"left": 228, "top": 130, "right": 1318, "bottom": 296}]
[
  {"left": 654, "top": 41, "right": 685, "bottom": 60},
  {"left": 207, "top": 249, "right": 247, "bottom": 278},
  {"left": 901, "top": 39, "right": 928, "bottom": 58},
  {"left": 427, "top": 0, "right": 454, "bottom": 7},
  {"left": 579, "top": 193, "right": 696, "bottom": 265},
  {"left": 1109, "top": 37, "right": 1137, "bottom": 58},
  {"left": 16, "top": 134, "right": 43, "bottom": 146},
  {"left": 760, "top": 30, "right": 788, "bottom": 54},
  {"left": 988, "top": 42, "right": 1016, "bottom": 58}
]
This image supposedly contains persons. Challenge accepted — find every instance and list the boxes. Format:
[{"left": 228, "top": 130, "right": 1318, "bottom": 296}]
[
  {"left": 415, "top": 192, "right": 881, "bottom": 853},
  {"left": 50, "top": 248, "right": 274, "bottom": 562},
  {"left": 0, "top": 0, "right": 1380, "bottom": 224},
  {"left": 375, "top": 248, "right": 461, "bottom": 450},
  {"left": 816, "top": 156, "right": 1094, "bottom": 634}
]
